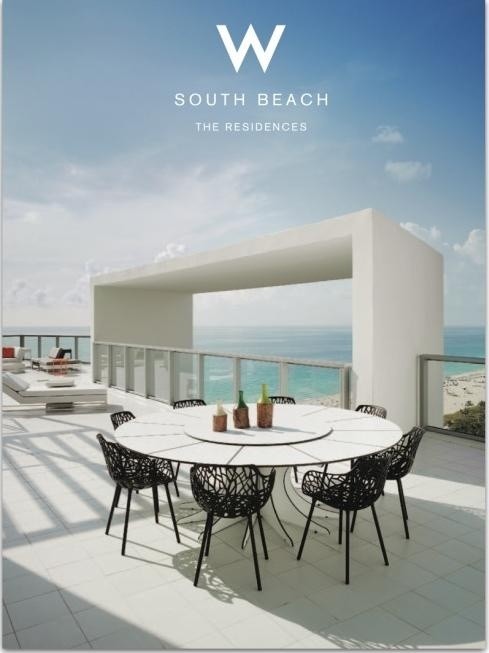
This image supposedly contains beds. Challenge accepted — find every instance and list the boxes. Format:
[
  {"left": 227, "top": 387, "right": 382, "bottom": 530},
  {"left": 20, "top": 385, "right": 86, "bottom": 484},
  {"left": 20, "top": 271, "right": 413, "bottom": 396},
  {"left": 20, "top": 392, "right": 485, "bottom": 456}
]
[{"left": 39, "top": 358, "right": 81, "bottom": 374}]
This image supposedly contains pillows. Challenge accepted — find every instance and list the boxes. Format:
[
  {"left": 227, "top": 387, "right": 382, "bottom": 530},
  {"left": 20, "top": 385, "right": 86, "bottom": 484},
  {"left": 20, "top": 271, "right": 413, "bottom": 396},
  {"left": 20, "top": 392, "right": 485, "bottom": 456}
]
[{"left": 45, "top": 377, "right": 75, "bottom": 387}]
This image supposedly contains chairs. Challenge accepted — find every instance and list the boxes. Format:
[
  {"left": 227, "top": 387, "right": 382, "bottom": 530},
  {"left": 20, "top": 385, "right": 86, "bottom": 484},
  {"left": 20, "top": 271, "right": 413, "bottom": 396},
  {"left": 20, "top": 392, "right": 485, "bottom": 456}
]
[
  {"left": 1, "top": 345, "right": 25, "bottom": 372},
  {"left": 2, "top": 371, "right": 107, "bottom": 414},
  {"left": 31, "top": 347, "right": 63, "bottom": 370},
  {"left": 2, "top": 347, "right": 15, "bottom": 358},
  {"left": 94, "top": 396, "right": 426, "bottom": 592}
]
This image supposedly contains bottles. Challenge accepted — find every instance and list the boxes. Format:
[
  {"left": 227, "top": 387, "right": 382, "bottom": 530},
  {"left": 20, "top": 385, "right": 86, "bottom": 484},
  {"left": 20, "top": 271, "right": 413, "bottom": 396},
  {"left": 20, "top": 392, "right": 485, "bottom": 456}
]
[
  {"left": 213, "top": 400, "right": 227, "bottom": 432},
  {"left": 257, "top": 384, "right": 273, "bottom": 427},
  {"left": 233, "top": 390, "right": 249, "bottom": 428}
]
